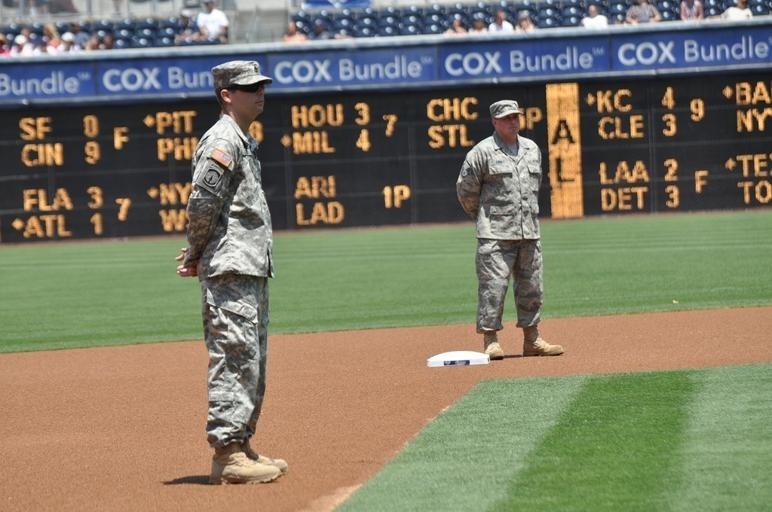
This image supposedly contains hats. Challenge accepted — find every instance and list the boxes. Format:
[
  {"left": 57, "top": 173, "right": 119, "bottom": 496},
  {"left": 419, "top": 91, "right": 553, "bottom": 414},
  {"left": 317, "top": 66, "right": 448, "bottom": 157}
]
[
  {"left": 210, "top": 60, "right": 273, "bottom": 87},
  {"left": 488, "top": 100, "right": 524, "bottom": 117}
]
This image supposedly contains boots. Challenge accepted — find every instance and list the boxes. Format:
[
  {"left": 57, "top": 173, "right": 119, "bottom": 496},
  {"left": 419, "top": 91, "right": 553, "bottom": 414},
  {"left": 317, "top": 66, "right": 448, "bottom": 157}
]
[
  {"left": 209, "top": 441, "right": 288, "bottom": 484},
  {"left": 483, "top": 332, "right": 504, "bottom": 359},
  {"left": 522, "top": 327, "right": 565, "bottom": 356}
]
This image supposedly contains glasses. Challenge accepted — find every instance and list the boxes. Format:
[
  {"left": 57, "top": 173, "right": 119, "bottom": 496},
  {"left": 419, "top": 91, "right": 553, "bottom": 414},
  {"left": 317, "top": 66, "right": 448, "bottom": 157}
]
[{"left": 229, "top": 86, "right": 265, "bottom": 93}]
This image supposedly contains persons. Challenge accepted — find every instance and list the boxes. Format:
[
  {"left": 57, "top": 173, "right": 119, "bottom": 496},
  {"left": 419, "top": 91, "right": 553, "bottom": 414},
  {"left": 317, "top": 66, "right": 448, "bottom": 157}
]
[
  {"left": 0, "top": 0, "right": 772, "bottom": 57},
  {"left": 456, "top": 96, "right": 566, "bottom": 360},
  {"left": 173, "top": 56, "right": 290, "bottom": 486}
]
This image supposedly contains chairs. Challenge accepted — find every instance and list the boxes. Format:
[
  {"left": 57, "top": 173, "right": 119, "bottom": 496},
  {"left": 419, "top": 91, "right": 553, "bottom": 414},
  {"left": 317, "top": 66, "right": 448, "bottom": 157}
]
[
  {"left": 291, "top": 0, "right": 772, "bottom": 40},
  {"left": 0, "top": 16, "right": 198, "bottom": 49}
]
